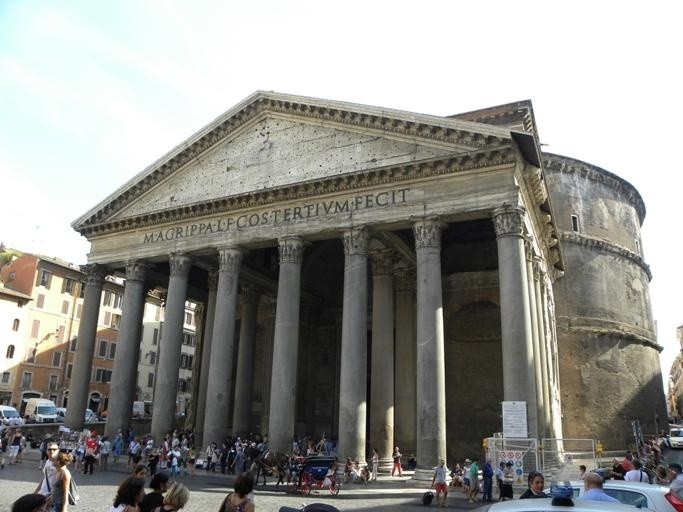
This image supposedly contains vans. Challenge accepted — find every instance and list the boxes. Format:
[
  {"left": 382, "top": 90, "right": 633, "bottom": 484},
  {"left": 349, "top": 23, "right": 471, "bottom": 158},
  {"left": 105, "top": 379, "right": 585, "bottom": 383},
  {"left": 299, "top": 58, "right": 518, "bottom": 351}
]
[{"left": 0, "top": 405, "right": 22, "bottom": 426}]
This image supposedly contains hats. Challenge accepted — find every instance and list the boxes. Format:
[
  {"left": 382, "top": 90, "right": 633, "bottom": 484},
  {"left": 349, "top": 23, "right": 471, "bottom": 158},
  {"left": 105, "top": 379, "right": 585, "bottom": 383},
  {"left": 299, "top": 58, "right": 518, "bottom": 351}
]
[{"left": 279, "top": 503, "right": 340, "bottom": 512}]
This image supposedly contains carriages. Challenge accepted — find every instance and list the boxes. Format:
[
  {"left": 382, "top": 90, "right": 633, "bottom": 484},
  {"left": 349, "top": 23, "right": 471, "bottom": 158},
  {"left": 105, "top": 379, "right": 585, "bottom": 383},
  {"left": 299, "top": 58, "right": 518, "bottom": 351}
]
[{"left": 242, "top": 442, "right": 340, "bottom": 496}]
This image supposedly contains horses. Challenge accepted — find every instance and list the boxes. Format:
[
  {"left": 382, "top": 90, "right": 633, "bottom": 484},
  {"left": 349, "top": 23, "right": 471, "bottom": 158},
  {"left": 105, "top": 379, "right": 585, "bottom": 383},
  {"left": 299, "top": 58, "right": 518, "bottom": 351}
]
[{"left": 242, "top": 444, "right": 290, "bottom": 490}]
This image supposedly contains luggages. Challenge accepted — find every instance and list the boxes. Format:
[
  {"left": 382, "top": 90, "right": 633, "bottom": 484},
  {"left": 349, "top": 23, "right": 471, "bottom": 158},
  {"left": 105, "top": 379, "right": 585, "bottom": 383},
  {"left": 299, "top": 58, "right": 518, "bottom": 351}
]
[{"left": 422, "top": 488, "right": 434, "bottom": 505}]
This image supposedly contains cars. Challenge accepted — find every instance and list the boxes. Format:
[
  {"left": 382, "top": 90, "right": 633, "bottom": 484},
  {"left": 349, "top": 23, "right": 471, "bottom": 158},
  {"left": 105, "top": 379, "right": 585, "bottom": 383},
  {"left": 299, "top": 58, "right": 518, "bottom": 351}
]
[
  {"left": 55, "top": 407, "right": 66, "bottom": 422},
  {"left": 666, "top": 427, "right": 683, "bottom": 449},
  {"left": 541, "top": 480, "right": 682, "bottom": 512},
  {"left": 84, "top": 408, "right": 98, "bottom": 422},
  {"left": 464, "top": 476, "right": 655, "bottom": 511}
]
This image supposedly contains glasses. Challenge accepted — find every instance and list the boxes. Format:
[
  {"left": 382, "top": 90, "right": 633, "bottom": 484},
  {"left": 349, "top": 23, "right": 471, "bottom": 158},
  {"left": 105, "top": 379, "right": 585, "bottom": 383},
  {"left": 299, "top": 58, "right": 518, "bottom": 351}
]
[{"left": 48, "top": 448, "right": 57, "bottom": 452}]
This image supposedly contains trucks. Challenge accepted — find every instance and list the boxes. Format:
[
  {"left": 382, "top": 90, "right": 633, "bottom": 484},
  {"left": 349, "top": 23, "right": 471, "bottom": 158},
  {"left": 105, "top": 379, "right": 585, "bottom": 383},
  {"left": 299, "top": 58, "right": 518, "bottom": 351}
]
[
  {"left": 131, "top": 401, "right": 152, "bottom": 418},
  {"left": 23, "top": 397, "right": 59, "bottom": 424}
]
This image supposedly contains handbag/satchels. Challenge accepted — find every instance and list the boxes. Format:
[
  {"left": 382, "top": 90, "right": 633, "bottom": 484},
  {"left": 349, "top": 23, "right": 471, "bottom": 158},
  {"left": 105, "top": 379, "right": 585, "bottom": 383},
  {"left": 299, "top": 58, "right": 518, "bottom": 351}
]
[{"left": 69, "top": 478, "right": 79, "bottom": 505}]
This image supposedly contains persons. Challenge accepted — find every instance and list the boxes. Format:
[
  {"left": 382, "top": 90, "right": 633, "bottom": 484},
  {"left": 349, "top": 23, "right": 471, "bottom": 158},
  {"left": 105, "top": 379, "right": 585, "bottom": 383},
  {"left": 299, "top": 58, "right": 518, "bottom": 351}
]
[
  {"left": 1, "top": 427, "right": 27, "bottom": 469},
  {"left": 32, "top": 441, "right": 59, "bottom": 507},
  {"left": 430, "top": 459, "right": 448, "bottom": 507},
  {"left": 392, "top": 447, "right": 402, "bottom": 477},
  {"left": 575, "top": 429, "right": 682, "bottom": 504},
  {"left": 48, "top": 452, "right": 71, "bottom": 512},
  {"left": 156, "top": 482, "right": 189, "bottom": 511},
  {"left": 206, "top": 437, "right": 268, "bottom": 485},
  {"left": 9, "top": 493, "right": 45, "bottom": 512},
  {"left": 343, "top": 448, "right": 379, "bottom": 485},
  {"left": 110, "top": 476, "right": 144, "bottom": 512},
  {"left": 139, "top": 474, "right": 170, "bottom": 511},
  {"left": 449, "top": 455, "right": 514, "bottom": 503},
  {"left": 218, "top": 471, "right": 255, "bottom": 511},
  {"left": 289, "top": 436, "right": 334, "bottom": 457},
  {"left": 519, "top": 470, "right": 549, "bottom": 498},
  {"left": 126, "top": 428, "right": 198, "bottom": 478},
  {"left": 38, "top": 427, "right": 124, "bottom": 474}
]
